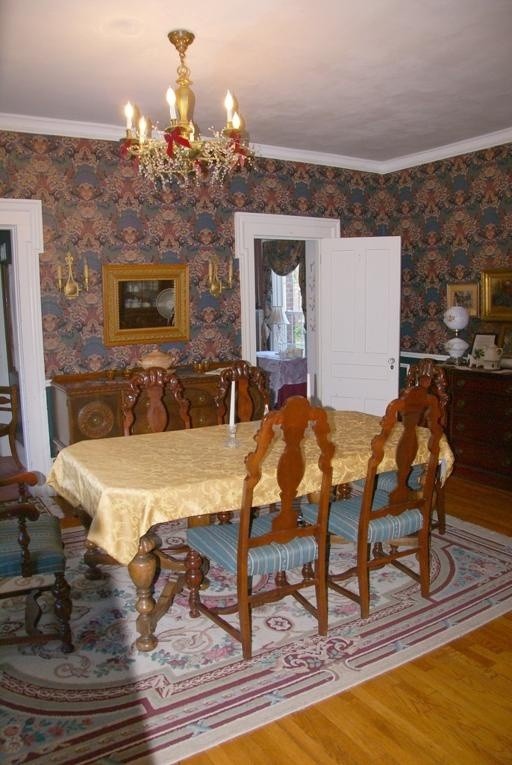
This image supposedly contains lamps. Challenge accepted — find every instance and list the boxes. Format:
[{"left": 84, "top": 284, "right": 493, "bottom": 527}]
[
  {"left": 203, "top": 254, "right": 233, "bottom": 297},
  {"left": 124, "top": 29, "right": 259, "bottom": 192},
  {"left": 57, "top": 248, "right": 91, "bottom": 297},
  {"left": 266, "top": 307, "right": 290, "bottom": 355},
  {"left": 443, "top": 306, "right": 468, "bottom": 360}
]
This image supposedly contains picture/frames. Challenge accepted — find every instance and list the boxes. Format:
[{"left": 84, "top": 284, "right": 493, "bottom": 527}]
[
  {"left": 446, "top": 280, "right": 481, "bottom": 320},
  {"left": 480, "top": 270, "right": 512, "bottom": 322},
  {"left": 469, "top": 332, "right": 497, "bottom": 368},
  {"left": 101, "top": 262, "right": 189, "bottom": 348}
]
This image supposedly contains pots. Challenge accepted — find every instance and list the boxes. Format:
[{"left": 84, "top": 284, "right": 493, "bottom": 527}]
[{"left": 138, "top": 349, "right": 176, "bottom": 371}]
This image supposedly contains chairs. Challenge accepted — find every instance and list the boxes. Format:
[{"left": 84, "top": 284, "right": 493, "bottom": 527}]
[
  {"left": 0, "top": 472, "right": 75, "bottom": 653},
  {"left": 351, "top": 358, "right": 447, "bottom": 538},
  {"left": 121, "top": 366, "right": 191, "bottom": 437},
  {"left": 0, "top": 385, "right": 26, "bottom": 471},
  {"left": 298, "top": 385, "right": 444, "bottom": 621},
  {"left": 215, "top": 361, "right": 269, "bottom": 427},
  {"left": 185, "top": 394, "right": 335, "bottom": 663}
]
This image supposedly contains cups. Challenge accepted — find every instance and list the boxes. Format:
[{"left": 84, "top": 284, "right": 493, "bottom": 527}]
[{"left": 280, "top": 343, "right": 303, "bottom": 359}]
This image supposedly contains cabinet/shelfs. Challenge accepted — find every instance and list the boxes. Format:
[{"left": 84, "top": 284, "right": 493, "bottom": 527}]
[
  {"left": 448, "top": 367, "right": 512, "bottom": 492},
  {"left": 50, "top": 355, "right": 267, "bottom": 459}
]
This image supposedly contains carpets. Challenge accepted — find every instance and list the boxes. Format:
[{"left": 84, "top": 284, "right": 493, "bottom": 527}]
[{"left": 0, "top": 522, "right": 512, "bottom": 765}]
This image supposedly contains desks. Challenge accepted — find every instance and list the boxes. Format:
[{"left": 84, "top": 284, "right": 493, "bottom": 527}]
[{"left": 256, "top": 350, "right": 307, "bottom": 405}]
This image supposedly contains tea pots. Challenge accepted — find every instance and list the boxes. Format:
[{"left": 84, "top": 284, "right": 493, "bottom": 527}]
[{"left": 483, "top": 344, "right": 503, "bottom": 370}]
